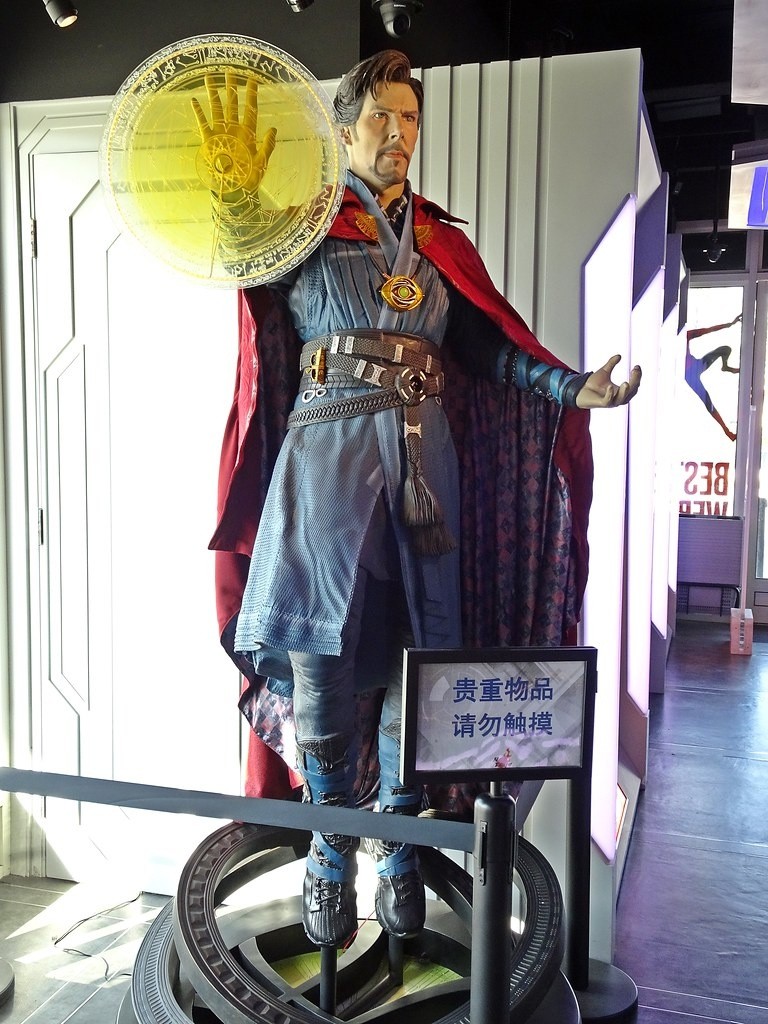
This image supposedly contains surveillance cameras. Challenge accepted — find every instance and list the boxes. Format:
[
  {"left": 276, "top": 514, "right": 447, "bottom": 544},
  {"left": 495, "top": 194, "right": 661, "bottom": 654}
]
[{"left": 372, "top": 0, "right": 424, "bottom": 38}]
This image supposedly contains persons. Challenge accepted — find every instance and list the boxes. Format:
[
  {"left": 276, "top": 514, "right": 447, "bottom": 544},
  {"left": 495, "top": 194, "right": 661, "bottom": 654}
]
[{"left": 192, "top": 45, "right": 641, "bottom": 947}]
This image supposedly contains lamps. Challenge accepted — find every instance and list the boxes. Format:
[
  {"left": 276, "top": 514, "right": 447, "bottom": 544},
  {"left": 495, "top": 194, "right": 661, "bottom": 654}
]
[
  {"left": 42, "top": 0, "right": 78, "bottom": 27},
  {"left": 287, "top": 0, "right": 314, "bottom": 13}
]
